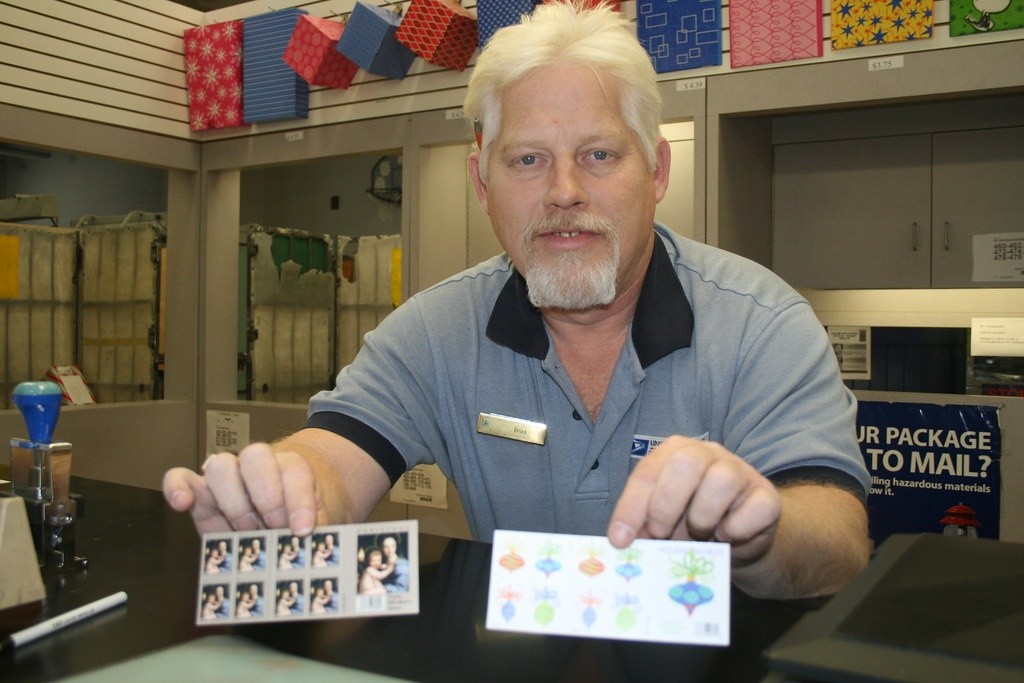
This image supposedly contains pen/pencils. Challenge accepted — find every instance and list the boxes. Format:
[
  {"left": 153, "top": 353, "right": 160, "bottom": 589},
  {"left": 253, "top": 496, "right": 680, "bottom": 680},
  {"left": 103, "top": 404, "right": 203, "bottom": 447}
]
[{"left": 0, "top": 591, "right": 127, "bottom": 655}]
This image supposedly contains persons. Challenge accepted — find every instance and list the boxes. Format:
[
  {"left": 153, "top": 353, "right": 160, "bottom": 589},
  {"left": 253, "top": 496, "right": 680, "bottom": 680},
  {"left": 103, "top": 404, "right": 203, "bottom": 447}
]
[
  {"left": 202, "top": 533, "right": 412, "bottom": 618},
  {"left": 158, "top": 1, "right": 874, "bottom": 603}
]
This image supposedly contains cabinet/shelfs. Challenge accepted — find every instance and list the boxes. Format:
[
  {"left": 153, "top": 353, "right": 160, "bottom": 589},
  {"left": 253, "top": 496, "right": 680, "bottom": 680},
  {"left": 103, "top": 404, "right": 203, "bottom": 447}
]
[{"left": 769, "top": 84, "right": 1024, "bottom": 289}]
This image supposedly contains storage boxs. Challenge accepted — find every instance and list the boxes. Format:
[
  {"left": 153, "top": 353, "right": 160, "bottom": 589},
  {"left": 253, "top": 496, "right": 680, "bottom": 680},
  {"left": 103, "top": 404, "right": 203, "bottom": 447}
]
[
  {"left": 393, "top": 0, "right": 479, "bottom": 72},
  {"left": 477, "top": 0, "right": 538, "bottom": 47},
  {"left": 245, "top": 8, "right": 308, "bottom": 123},
  {"left": 336, "top": 0, "right": 418, "bottom": 81},
  {"left": 281, "top": 14, "right": 360, "bottom": 89},
  {"left": 184, "top": 16, "right": 252, "bottom": 132}
]
